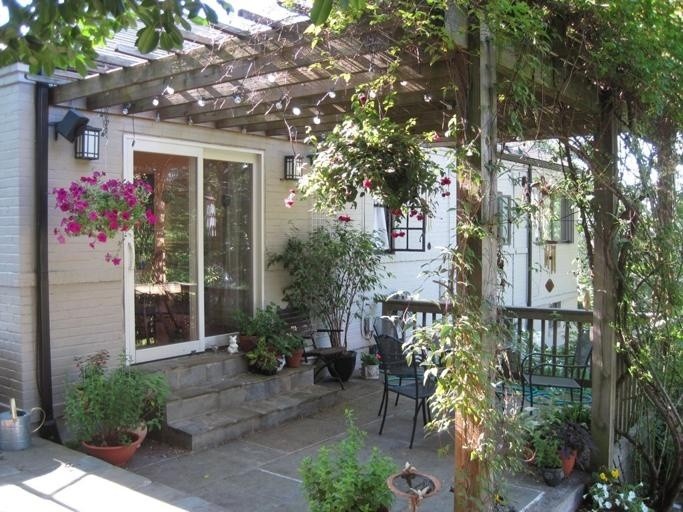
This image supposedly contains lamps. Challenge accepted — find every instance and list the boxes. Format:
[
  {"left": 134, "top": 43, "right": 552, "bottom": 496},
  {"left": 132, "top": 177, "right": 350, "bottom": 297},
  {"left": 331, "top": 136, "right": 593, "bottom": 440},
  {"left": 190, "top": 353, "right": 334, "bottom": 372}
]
[
  {"left": 282, "top": 155, "right": 303, "bottom": 183},
  {"left": 73, "top": 121, "right": 105, "bottom": 162}
]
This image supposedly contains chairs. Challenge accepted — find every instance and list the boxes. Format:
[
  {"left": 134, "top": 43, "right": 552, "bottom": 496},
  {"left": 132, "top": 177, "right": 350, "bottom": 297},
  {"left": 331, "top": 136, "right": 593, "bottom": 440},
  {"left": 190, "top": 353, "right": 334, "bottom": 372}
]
[{"left": 370, "top": 311, "right": 598, "bottom": 448}]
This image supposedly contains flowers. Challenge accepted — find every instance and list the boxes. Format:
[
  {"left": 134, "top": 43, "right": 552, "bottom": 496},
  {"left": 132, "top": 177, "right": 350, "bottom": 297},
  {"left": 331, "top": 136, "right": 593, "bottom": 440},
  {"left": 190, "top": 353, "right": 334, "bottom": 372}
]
[
  {"left": 65, "top": 348, "right": 167, "bottom": 441},
  {"left": 283, "top": 91, "right": 452, "bottom": 239}
]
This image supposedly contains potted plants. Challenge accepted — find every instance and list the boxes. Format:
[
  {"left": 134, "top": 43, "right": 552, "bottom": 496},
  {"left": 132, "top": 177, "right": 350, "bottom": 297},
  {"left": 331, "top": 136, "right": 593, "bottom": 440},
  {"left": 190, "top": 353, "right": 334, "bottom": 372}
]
[
  {"left": 238, "top": 223, "right": 386, "bottom": 388},
  {"left": 516, "top": 407, "right": 591, "bottom": 487}
]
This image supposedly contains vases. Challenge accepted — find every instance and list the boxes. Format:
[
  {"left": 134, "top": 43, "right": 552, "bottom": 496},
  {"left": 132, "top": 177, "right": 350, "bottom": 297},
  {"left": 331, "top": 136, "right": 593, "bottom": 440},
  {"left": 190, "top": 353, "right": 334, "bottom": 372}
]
[{"left": 78, "top": 430, "right": 144, "bottom": 465}]
